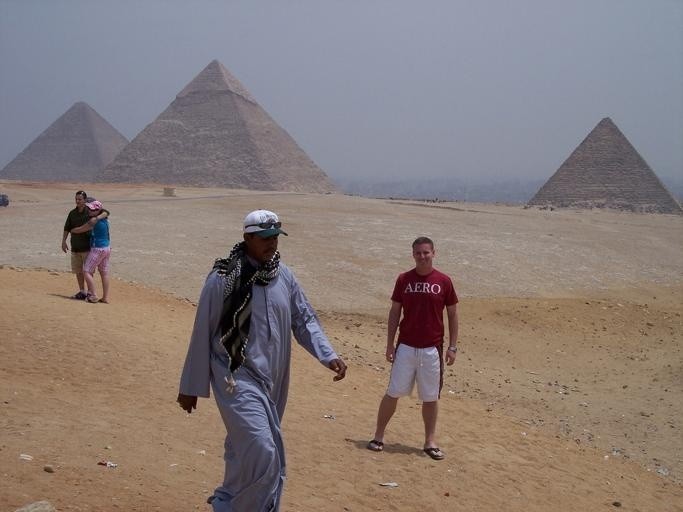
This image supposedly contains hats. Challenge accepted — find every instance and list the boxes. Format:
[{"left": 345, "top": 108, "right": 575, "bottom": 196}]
[
  {"left": 243, "top": 210, "right": 288, "bottom": 239},
  {"left": 86, "top": 201, "right": 103, "bottom": 210}
]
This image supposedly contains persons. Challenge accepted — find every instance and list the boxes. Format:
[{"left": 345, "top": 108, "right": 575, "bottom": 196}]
[
  {"left": 62, "top": 191, "right": 110, "bottom": 300},
  {"left": 70, "top": 201, "right": 111, "bottom": 303},
  {"left": 369, "top": 237, "right": 459, "bottom": 460},
  {"left": 176, "top": 210, "right": 347, "bottom": 512}
]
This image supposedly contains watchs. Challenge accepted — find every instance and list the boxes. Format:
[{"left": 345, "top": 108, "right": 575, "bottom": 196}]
[{"left": 448, "top": 346, "right": 458, "bottom": 353}]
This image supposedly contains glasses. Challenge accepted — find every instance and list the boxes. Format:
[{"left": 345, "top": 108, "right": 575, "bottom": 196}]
[{"left": 246, "top": 222, "right": 282, "bottom": 229}]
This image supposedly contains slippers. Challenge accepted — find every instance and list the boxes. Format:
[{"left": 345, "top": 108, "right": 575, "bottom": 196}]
[
  {"left": 424, "top": 448, "right": 444, "bottom": 460},
  {"left": 368, "top": 440, "right": 384, "bottom": 451},
  {"left": 72, "top": 292, "right": 110, "bottom": 304}
]
